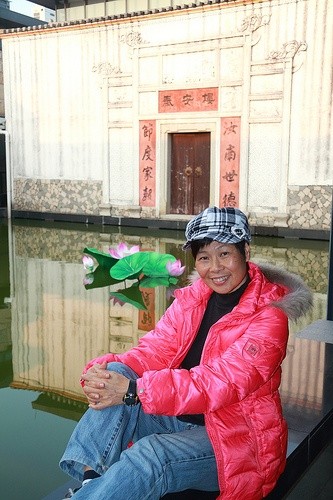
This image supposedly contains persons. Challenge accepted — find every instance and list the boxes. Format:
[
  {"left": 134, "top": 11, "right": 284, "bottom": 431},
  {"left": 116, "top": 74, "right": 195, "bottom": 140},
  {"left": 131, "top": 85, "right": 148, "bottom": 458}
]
[{"left": 58, "top": 205, "right": 315, "bottom": 500}]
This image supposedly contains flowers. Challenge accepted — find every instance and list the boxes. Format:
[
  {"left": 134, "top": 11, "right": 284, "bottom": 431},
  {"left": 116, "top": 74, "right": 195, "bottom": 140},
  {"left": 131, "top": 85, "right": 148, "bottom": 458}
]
[{"left": 80, "top": 240, "right": 186, "bottom": 313}]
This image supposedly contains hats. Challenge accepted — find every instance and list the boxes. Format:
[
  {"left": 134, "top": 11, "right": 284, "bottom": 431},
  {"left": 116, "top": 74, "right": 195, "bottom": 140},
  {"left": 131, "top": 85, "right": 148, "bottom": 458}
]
[{"left": 181, "top": 206, "right": 250, "bottom": 251}]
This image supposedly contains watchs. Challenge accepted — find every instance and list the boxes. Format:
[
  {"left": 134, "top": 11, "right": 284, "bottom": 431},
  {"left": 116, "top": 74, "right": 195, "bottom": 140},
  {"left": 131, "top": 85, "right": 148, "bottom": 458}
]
[{"left": 122, "top": 379, "right": 139, "bottom": 407}]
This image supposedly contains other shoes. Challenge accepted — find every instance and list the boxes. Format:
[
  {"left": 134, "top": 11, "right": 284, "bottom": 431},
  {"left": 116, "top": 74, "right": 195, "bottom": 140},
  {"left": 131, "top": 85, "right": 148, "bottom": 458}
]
[{"left": 63, "top": 480, "right": 97, "bottom": 498}]
